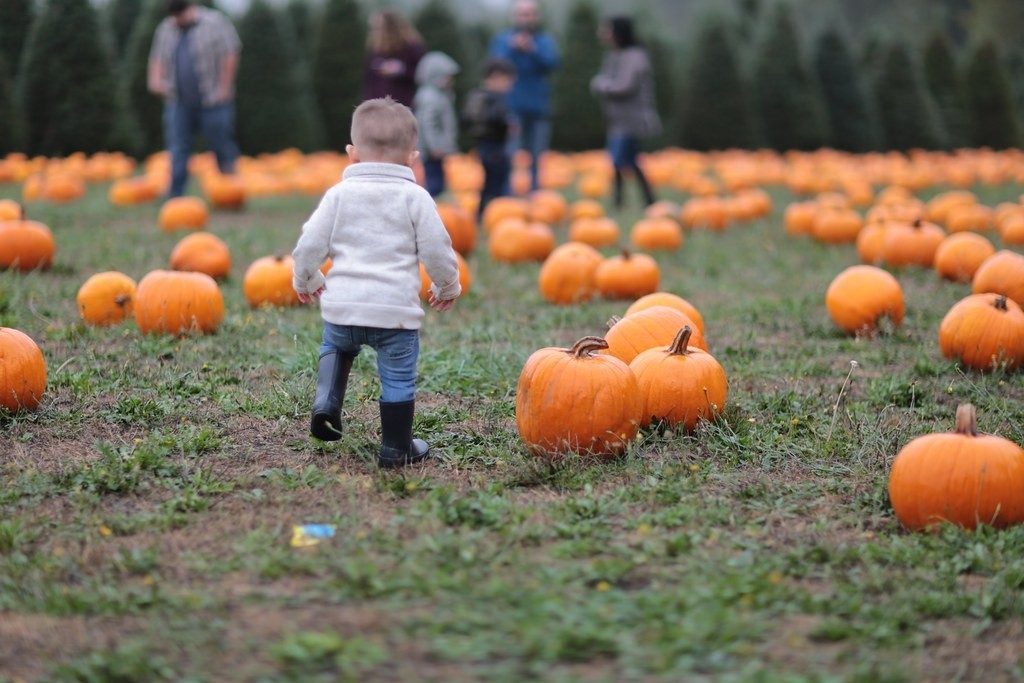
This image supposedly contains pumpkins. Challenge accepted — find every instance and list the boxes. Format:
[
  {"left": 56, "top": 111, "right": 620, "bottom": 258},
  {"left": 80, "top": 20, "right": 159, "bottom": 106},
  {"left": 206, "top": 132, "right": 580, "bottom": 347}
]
[
  {"left": 888, "top": 401, "right": 1024, "bottom": 535},
  {"left": 516, "top": 336, "right": 642, "bottom": 461},
  {"left": 629, "top": 325, "right": 726, "bottom": 434},
  {"left": 410, "top": 144, "right": 1024, "bottom": 370},
  {"left": 0, "top": 147, "right": 351, "bottom": 336},
  {"left": 0, "top": 326, "right": 46, "bottom": 415}
]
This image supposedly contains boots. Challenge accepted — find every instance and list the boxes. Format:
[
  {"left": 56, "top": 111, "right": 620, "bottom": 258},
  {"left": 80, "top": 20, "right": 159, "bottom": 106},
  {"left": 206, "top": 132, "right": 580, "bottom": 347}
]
[
  {"left": 628, "top": 161, "right": 658, "bottom": 210},
  {"left": 310, "top": 349, "right": 355, "bottom": 441},
  {"left": 378, "top": 394, "right": 430, "bottom": 468},
  {"left": 609, "top": 161, "right": 626, "bottom": 210}
]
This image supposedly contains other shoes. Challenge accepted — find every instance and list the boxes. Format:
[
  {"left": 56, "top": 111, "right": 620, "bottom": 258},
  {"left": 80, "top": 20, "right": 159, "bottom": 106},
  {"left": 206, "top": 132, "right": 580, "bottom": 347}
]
[{"left": 156, "top": 187, "right": 186, "bottom": 206}]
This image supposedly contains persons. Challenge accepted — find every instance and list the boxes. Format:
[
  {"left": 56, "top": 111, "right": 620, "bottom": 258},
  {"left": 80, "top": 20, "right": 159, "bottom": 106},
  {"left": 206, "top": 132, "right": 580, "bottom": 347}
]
[
  {"left": 146, "top": 1, "right": 242, "bottom": 204},
  {"left": 292, "top": 97, "right": 461, "bottom": 468},
  {"left": 365, "top": 0, "right": 663, "bottom": 225}
]
